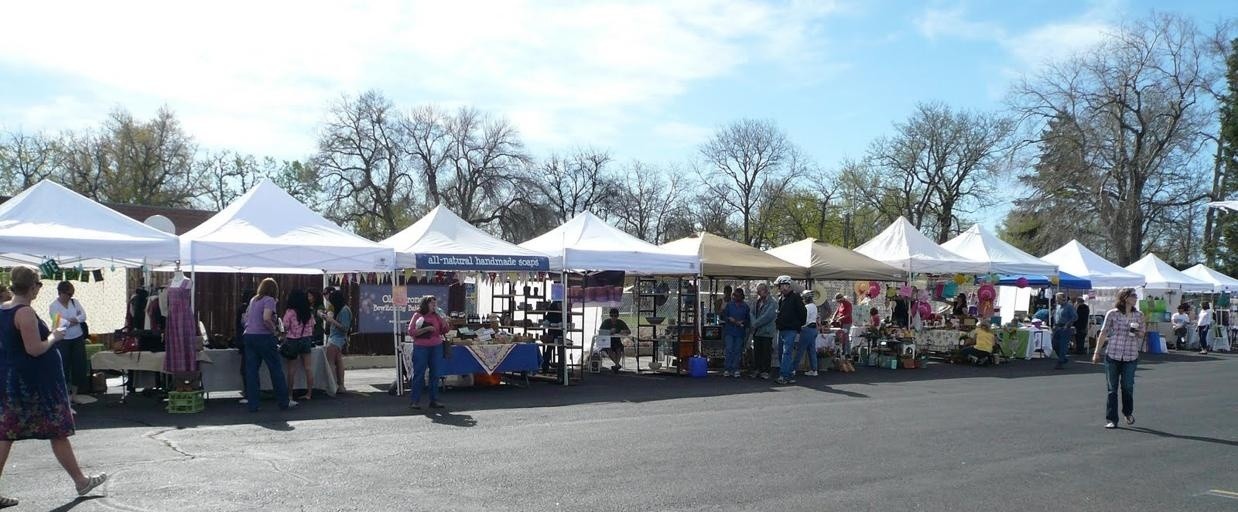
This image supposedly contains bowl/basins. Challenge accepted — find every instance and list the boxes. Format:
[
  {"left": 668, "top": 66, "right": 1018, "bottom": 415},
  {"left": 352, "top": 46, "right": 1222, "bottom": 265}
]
[
  {"left": 551, "top": 322, "right": 558, "bottom": 327},
  {"left": 646, "top": 317, "right": 666, "bottom": 324},
  {"left": 649, "top": 362, "right": 662, "bottom": 369}
]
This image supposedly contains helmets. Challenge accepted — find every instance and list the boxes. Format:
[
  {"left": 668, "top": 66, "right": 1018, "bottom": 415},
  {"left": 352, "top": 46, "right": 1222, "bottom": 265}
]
[
  {"left": 799, "top": 289, "right": 815, "bottom": 299},
  {"left": 773, "top": 275, "right": 792, "bottom": 285}
]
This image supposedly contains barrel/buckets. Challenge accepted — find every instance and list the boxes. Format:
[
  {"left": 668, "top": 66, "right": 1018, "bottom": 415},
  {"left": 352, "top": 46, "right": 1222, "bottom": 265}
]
[
  {"left": 1145, "top": 331, "right": 1161, "bottom": 354},
  {"left": 687, "top": 354, "right": 708, "bottom": 378}
]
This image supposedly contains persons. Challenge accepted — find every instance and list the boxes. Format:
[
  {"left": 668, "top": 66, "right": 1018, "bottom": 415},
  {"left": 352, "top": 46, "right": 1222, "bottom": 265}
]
[
  {"left": 1092, "top": 287, "right": 1146, "bottom": 428},
  {"left": 408, "top": 294, "right": 449, "bottom": 410},
  {"left": 47, "top": 280, "right": 88, "bottom": 403},
  {"left": 718, "top": 276, "right": 853, "bottom": 385},
  {"left": 1170, "top": 295, "right": 1213, "bottom": 356},
  {"left": 0, "top": 284, "right": 12, "bottom": 302},
  {"left": 235, "top": 275, "right": 351, "bottom": 413},
  {"left": 600, "top": 308, "right": 631, "bottom": 373},
  {"left": 868, "top": 308, "right": 880, "bottom": 325},
  {"left": 943, "top": 292, "right": 970, "bottom": 321},
  {"left": 1035, "top": 291, "right": 1088, "bottom": 370},
  {"left": 961, "top": 321, "right": 996, "bottom": 365},
  {"left": 162, "top": 269, "right": 196, "bottom": 374},
  {"left": 543, "top": 300, "right": 570, "bottom": 369},
  {"left": 0, "top": 267, "right": 108, "bottom": 507}
]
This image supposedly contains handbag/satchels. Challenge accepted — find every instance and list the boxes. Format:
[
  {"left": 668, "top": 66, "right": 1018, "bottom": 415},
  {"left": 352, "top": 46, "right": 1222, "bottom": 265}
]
[
  {"left": 111, "top": 325, "right": 204, "bottom": 354},
  {"left": 441, "top": 340, "right": 452, "bottom": 360},
  {"left": 806, "top": 322, "right": 817, "bottom": 328},
  {"left": 279, "top": 338, "right": 301, "bottom": 361},
  {"left": 80, "top": 321, "right": 89, "bottom": 339},
  {"left": 343, "top": 336, "right": 352, "bottom": 356},
  {"left": 415, "top": 322, "right": 434, "bottom": 338}
]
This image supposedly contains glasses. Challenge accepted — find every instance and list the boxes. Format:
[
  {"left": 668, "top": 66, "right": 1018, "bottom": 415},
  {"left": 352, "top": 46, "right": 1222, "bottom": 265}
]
[
  {"left": 1127, "top": 293, "right": 1137, "bottom": 298},
  {"left": 34, "top": 280, "right": 43, "bottom": 287}
]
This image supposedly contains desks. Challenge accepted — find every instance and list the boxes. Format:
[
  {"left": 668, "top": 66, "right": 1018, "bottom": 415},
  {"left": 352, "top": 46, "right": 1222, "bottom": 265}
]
[
  {"left": 402, "top": 341, "right": 542, "bottom": 394},
  {"left": 89, "top": 343, "right": 338, "bottom": 399},
  {"left": 889, "top": 326, "right": 972, "bottom": 360},
  {"left": 1003, "top": 326, "right": 1053, "bottom": 360}
]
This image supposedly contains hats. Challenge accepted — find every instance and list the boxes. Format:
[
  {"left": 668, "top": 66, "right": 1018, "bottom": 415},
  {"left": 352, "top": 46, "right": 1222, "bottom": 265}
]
[
  {"left": 810, "top": 284, "right": 828, "bottom": 307},
  {"left": 831, "top": 292, "right": 848, "bottom": 303},
  {"left": 911, "top": 285, "right": 932, "bottom": 321},
  {"left": 977, "top": 284, "right": 997, "bottom": 305},
  {"left": 854, "top": 281, "right": 881, "bottom": 299}
]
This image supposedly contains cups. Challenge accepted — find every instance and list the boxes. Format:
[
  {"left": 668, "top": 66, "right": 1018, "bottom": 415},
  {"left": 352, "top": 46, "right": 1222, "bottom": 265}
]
[
  {"left": 91, "top": 332, "right": 98, "bottom": 345},
  {"left": 667, "top": 318, "right": 676, "bottom": 325},
  {"left": 1128, "top": 321, "right": 1140, "bottom": 339}
]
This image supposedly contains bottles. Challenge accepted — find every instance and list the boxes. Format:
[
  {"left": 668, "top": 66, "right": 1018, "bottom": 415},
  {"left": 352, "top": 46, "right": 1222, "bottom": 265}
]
[
  {"left": 450, "top": 311, "right": 491, "bottom": 324},
  {"left": 542, "top": 319, "right": 549, "bottom": 328},
  {"left": 975, "top": 317, "right": 984, "bottom": 327},
  {"left": 1022, "top": 315, "right": 1031, "bottom": 326},
  {"left": 433, "top": 306, "right": 445, "bottom": 318},
  {"left": 514, "top": 332, "right": 534, "bottom": 343},
  {"left": 326, "top": 311, "right": 335, "bottom": 326}
]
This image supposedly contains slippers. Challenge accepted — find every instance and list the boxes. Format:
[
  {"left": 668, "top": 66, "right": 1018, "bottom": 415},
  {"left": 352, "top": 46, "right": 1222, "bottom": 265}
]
[
  {"left": 238, "top": 386, "right": 348, "bottom": 411},
  {"left": 0, "top": 494, "right": 18, "bottom": 509},
  {"left": 76, "top": 472, "right": 107, "bottom": 496},
  {"left": 1123, "top": 414, "right": 1136, "bottom": 425},
  {"left": 1103, "top": 421, "right": 1119, "bottom": 429}
]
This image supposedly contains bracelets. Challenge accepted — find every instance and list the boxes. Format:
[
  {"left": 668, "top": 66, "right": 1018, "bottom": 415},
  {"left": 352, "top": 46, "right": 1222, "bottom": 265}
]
[{"left": 1093, "top": 352, "right": 1100, "bottom": 354}]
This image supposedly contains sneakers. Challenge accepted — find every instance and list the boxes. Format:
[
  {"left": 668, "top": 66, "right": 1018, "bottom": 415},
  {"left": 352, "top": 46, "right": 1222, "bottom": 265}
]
[
  {"left": 428, "top": 400, "right": 445, "bottom": 409},
  {"left": 175, "top": 384, "right": 193, "bottom": 392},
  {"left": 1054, "top": 358, "right": 1069, "bottom": 370},
  {"left": 722, "top": 368, "right": 819, "bottom": 385},
  {"left": 409, "top": 401, "right": 422, "bottom": 410},
  {"left": 611, "top": 362, "right": 622, "bottom": 375}
]
